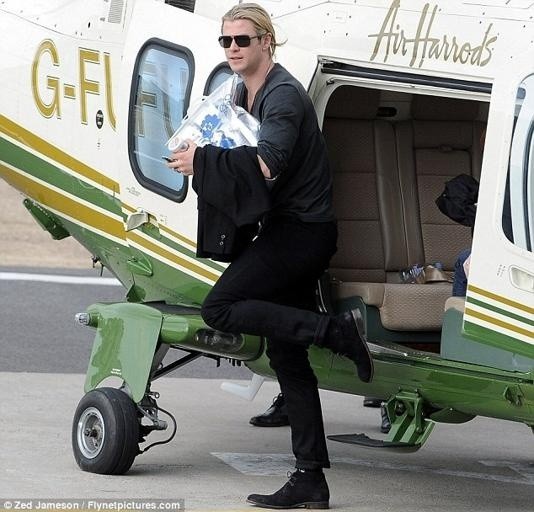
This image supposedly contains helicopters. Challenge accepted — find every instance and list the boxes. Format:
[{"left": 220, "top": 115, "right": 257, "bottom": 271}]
[{"left": 0, "top": 0, "right": 534, "bottom": 480}]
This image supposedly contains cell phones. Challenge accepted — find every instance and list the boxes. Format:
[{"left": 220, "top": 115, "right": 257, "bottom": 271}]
[{"left": 161, "top": 155, "right": 179, "bottom": 169}]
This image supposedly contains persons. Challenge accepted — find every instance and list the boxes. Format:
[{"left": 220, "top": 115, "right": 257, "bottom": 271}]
[
  {"left": 163, "top": 3, "right": 374, "bottom": 512},
  {"left": 248, "top": 388, "right": 294, "bottom": 429}
]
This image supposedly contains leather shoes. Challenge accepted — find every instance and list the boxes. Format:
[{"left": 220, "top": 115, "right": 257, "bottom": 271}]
[
  {"left": 378, "top": 404, "right": 390, "bottom": 435},
  {"left": 245, "top": 393, "right": 292, "bottom": 428},
  {"left": 321, "top": 306, "right": 376, "bottom": 385},
  {"left": 244, "top": 467, "right": 331, "bottom": 511}
]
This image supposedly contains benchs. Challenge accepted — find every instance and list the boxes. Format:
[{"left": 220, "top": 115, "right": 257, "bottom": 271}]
[{"left": 322, "top": 85, "right": 489, "bottom": 331}]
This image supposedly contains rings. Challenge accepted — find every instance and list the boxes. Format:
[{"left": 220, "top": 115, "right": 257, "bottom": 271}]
[{"left": 174, "top": 168, "right": 181, "bottom": 173}]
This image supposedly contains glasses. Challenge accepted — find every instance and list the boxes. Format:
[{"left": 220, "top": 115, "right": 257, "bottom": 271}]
[{"left": 217, "top": 34, "right": 267, "bottom": 49}]
